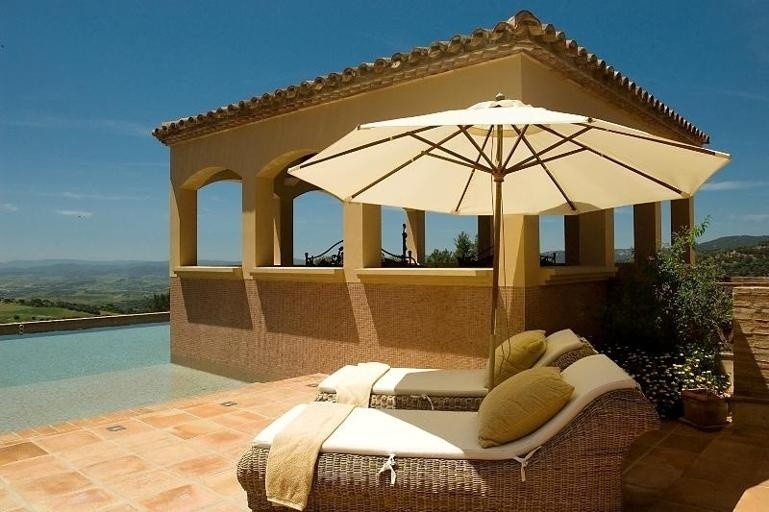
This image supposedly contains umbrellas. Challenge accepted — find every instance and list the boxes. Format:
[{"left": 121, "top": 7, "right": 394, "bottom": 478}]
[{"left": 286, "top": 93, "right": 736, "bottom": 395}]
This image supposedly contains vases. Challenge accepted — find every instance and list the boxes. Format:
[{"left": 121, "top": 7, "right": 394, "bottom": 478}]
[{"left": 679, "top": 388, "right": 731, "bottom": 431}]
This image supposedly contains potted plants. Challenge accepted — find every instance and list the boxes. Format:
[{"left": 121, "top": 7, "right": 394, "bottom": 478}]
[{"left": 673, "top": 349, "right": 734, "bottom": 399}]
